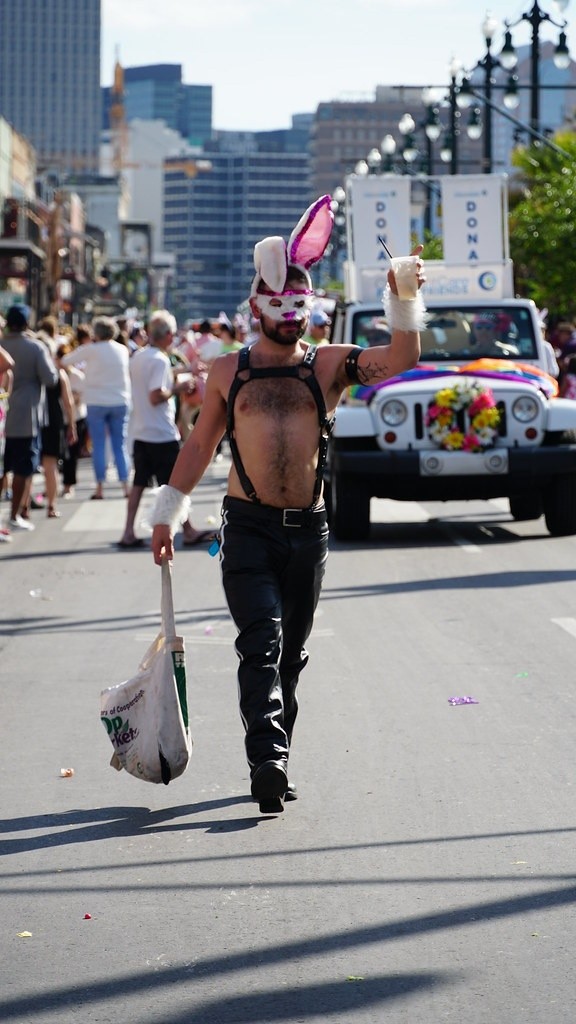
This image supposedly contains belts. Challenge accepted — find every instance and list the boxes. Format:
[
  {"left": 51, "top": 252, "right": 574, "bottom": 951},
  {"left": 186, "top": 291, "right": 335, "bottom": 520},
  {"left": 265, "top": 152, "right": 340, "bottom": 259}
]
[{"left": 223, "top": 495, "right": 326, "bottom": 527}]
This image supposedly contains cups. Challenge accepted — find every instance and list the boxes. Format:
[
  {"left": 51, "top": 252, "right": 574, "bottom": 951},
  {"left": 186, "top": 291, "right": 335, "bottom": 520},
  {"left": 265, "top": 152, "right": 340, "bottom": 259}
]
[{"left": 390, "top": 255, "right": 420, "bottom": 301}]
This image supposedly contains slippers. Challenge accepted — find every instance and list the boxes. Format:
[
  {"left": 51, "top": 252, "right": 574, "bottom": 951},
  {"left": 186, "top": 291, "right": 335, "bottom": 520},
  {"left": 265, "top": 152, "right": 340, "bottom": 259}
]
[
  {"left": 183, "top": 531, "right": 217, "bottom": 544},
  {"left": 118, "top": 539, "right": 146, "bottom": 548}
]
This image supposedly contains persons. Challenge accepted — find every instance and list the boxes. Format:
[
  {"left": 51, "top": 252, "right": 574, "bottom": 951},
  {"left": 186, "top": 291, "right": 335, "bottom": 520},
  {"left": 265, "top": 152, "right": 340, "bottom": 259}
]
[
  {"left": 152, "top": 243, "right": 425, "bottom": 814},
  {"left": 367, "top": 324, "right": 391, "bottom": 348},
  {"left": 462, "top": 313, "right": 521, "bottom": 356},
  {"left": 0, "top": 307, "right": 334, "bottom": 556},
  {"left": 539, "top": 321, "right": 576, "bottom": 400}
]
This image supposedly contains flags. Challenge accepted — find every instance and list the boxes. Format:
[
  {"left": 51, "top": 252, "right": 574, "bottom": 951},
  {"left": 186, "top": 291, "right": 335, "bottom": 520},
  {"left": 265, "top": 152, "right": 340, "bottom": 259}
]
[
  {"left": 352, "top": 175, "right": 413, "bottom": 263},
  {"left": 440, "top": 174, "right": 505, "bottom": 264}
]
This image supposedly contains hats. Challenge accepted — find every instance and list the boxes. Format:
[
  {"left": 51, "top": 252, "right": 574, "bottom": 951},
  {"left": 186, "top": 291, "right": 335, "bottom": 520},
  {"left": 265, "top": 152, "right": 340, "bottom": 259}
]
[{"left": 6, "top": 303, "right": 30, "bottom": 324}]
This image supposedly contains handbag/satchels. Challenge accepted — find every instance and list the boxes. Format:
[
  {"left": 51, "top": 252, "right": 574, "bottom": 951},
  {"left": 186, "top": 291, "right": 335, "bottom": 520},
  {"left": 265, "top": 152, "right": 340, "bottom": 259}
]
[{"left": 101, "top": 554, "right": 191, "bottom": 785}]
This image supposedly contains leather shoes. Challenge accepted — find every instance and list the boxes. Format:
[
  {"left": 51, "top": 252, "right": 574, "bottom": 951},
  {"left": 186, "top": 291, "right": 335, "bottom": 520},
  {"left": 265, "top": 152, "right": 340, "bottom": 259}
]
[
  {"left": 252, "top": 761, "right": 284, "bottom": 811},
  {"left": 286, "top": 782, "right": 297, "bottom": 800}
]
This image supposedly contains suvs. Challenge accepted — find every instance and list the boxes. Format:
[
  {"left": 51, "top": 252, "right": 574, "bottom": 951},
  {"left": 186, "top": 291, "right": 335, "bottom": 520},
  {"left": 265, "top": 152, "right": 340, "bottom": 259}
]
[{"left": 313, "top": 296, "right": 576, "bottom": 537}]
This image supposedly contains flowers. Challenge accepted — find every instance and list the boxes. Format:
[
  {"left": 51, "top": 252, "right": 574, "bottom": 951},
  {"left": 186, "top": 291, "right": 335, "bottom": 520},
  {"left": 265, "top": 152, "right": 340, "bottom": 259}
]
[{"left": 426, "top": 380, "right": 503, "bottom": 456}]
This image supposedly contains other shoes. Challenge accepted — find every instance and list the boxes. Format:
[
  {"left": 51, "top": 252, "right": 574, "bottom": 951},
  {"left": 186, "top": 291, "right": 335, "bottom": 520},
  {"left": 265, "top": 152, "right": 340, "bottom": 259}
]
[
  {"left": 90, "top": 495, "right": 102, "bottom": 499},
  {"left": 60, "top": 492, "right": 74, "bottom": 499},
  {"left": 9, "top": 515, "right": 33, "bottom": 531},
  {"left": 0, "top": 531, "right": 12, "bottom": 542},
  {"left": 48, "top": 510, "right": 61, "bottom": 517},
  {"left": 23, "top": 514, "right": 29, "bottom": 519}
]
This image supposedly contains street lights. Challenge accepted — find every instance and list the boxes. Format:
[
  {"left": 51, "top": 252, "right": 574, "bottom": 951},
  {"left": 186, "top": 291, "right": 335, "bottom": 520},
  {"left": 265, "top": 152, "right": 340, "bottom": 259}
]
[{"left": 316, "top": 0, "right": 576, "bottom": 290}]
